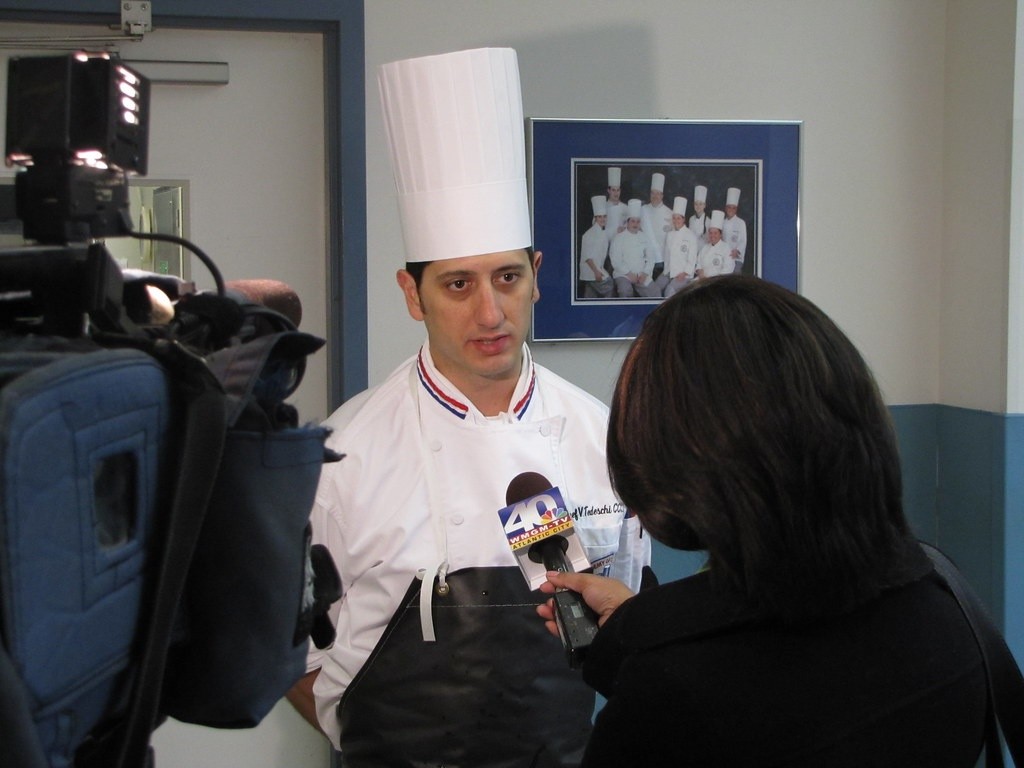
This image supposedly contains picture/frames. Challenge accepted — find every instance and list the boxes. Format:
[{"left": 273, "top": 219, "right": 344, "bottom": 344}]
[{"left": 526, "top": 116, "right": 805, "bottom": 341}]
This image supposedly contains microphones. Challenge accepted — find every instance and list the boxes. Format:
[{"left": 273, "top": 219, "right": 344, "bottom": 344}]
[
  {"left": 175, "top": 279, "right": 302, "bottom": 350},
  {"left": 497, "top": 471, "right": 599, "bottom": 652}
]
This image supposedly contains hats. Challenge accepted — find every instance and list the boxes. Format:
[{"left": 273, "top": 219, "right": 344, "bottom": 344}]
[
  {"left": 628, "top": 199, "right": 641, "bottom": 217},
  {"left": 651, "top": 173, "right": 664, "bottom": 193},
  {"left": 708, "top": 210, "right": 725, "bottom": 232},
  {"left": 374, "top": 47, "right": 532, "bottom": 263},
  {"left": 607, "top": 167, "right": 621, "bottom": 186},
  {"left": 694, "top": 185, "right": 708, "bottom": 203},
  {"left": 726, "top": 187, "right": 741, "bottom": 207},
  {"left": 673, "top": 196, "right": 687, "bottom": 218},
  {"left": 591, "top": 195, "right": 608, "bottom": 216}
]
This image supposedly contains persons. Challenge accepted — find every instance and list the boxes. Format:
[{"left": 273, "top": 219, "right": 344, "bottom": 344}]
[
  {"left": 284, "top": 46, "right": 652, "bottom": 768},
  {"left": 581, "top": 167, "right": 748, "bottom": 299},
  {"left": 536, "top": 273, "right": 1023, "bottom": 768}
]
